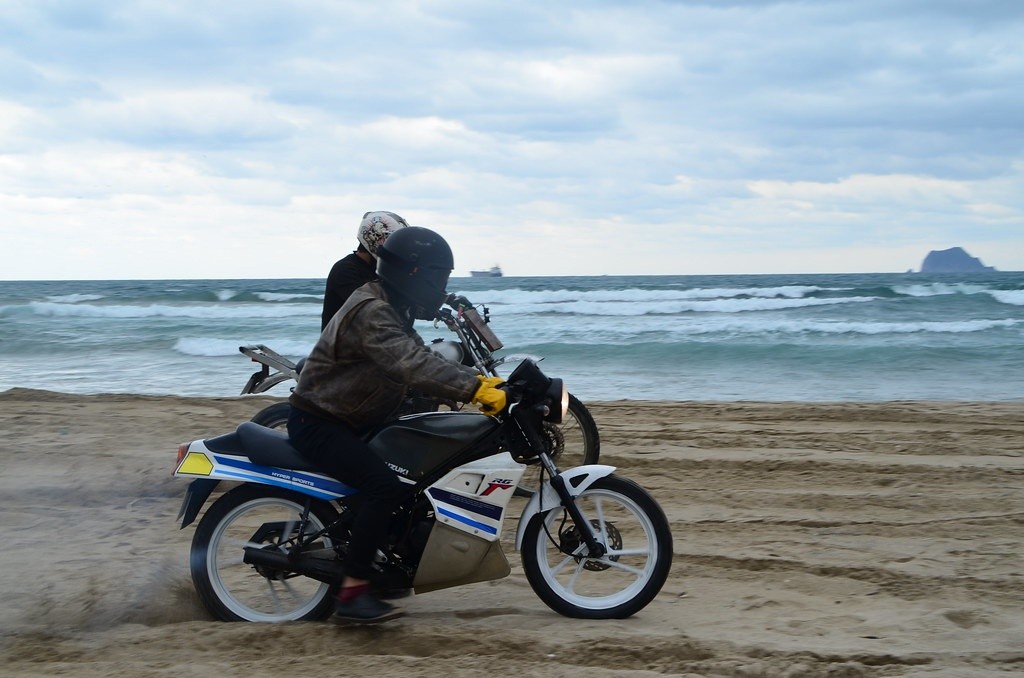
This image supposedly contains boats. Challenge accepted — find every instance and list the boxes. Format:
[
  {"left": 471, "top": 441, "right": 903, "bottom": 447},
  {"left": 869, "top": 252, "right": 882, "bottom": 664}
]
[{"left": 471, "top": 264, "right": 502, "bottom": 278}]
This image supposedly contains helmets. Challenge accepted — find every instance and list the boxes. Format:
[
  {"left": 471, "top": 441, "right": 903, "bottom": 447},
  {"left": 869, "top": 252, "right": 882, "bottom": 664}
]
[
  {"left": 357, "top": 211, "right": 410, "bottom": 261},
  {"left": 376, "top": 226, "right": 454, "bottom": 314}
]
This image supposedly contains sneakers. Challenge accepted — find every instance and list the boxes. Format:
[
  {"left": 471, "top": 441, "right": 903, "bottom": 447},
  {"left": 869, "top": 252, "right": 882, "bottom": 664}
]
[{"left": 332, "top": 593, "right": 406, "bottom": 624}]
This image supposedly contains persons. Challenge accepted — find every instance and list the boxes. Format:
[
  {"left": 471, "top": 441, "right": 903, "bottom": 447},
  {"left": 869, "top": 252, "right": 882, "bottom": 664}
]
[
  {"left": 287, "top": 226, "right": 508, "bottom": 622},
  {"left": 320, "top": 211, "right": 410, "bottom": 334}
]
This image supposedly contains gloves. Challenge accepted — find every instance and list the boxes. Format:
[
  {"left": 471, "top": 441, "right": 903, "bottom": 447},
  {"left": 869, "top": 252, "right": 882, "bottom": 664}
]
[
  {"left": 445, "top": 292, "right": 472, "bottom": 311},
  {"left": 472, "top": 375, "right": 506, "bottom": 416}
]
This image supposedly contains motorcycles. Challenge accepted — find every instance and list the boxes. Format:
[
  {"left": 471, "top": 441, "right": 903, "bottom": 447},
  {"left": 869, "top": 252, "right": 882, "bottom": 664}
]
[
  {"left": 170, "top": 359, "right": 674, "bottom": 622},
  {"left": 239, "top": 303, "right": 601, "bottom": 499}
]
